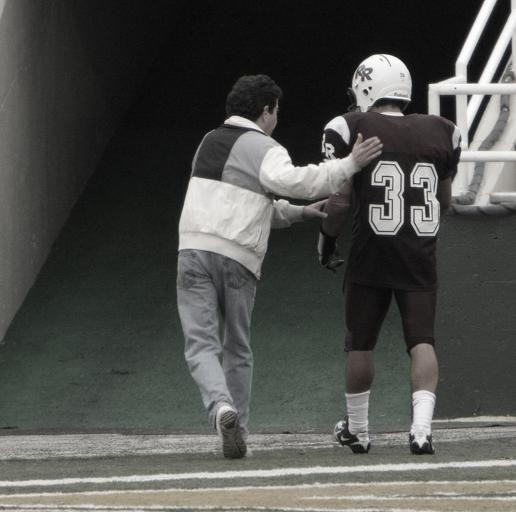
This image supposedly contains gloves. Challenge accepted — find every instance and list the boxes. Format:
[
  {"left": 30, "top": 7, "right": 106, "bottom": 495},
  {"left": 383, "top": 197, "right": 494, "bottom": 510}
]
[{"left": 318, "top": 229, "right": 345, "bottom": 272}]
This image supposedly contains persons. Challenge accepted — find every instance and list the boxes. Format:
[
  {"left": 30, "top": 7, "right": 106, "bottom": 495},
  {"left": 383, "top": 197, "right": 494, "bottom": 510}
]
[
  {"left": 312, "top": 54, "right": 466, "bottom": 456},
  {"left": 174, "top": 73, "right": 384, "bottom": 461}
]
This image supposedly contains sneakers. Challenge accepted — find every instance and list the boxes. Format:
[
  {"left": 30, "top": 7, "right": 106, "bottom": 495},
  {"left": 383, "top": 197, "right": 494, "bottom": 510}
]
[
  {"left": 408, "top": 432, "right": 435, "bottom": 453},
  {"left": 334, "top": 421, "right": 370, "bottom": 453},
  {"left": 215, "top": 406, "right": 248, "bottom": 457}
]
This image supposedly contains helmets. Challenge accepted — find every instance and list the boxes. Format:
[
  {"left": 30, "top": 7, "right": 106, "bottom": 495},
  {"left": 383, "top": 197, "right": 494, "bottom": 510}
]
[{"left": 347, "top": 54, "right": 412, "bottom": 113}]
